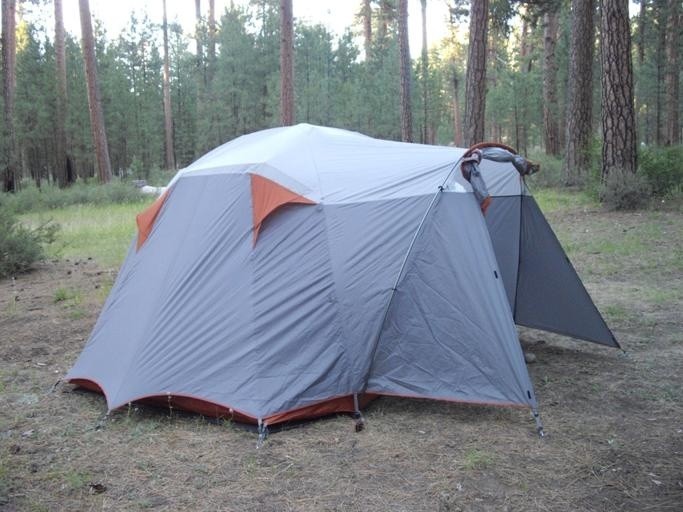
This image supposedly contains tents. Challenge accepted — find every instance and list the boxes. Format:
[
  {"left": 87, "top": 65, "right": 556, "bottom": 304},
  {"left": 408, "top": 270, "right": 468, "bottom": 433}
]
[{"left": 65, "top": 123, "right": 626, "bottom": 436}]
